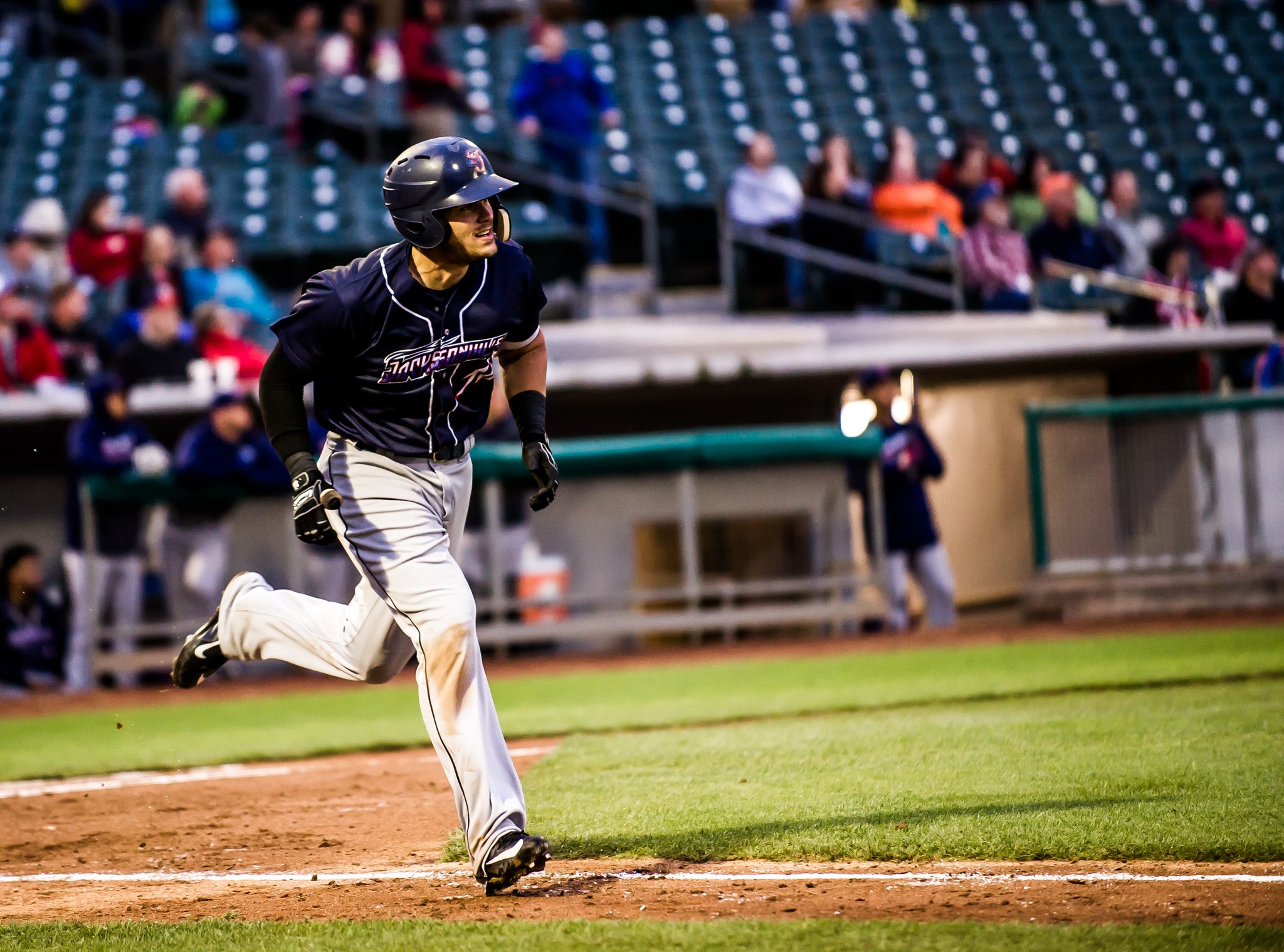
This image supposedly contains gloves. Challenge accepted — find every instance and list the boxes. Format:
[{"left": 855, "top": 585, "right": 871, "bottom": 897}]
[
  {"left": 290, "top": 459, "right": 344, "bottom": 547},
  {"left": 520, "top": 431, "right": 558, "bottom": 514}
]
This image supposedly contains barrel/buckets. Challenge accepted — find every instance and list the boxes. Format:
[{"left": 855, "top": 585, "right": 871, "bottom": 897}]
[{"left": 517, "top": 551, "right": 574, "bottom": 624}]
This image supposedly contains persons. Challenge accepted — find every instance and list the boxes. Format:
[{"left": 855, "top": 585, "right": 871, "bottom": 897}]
[
  {"left": 0, "top": 161, "right": 352, "bottom": 696},
  {"left": 845, "top": 369, "right": 959, "bottom": 630},
  {"left": 128, "top": 1, "right": 496, "bottom": 155},
  {"left": 720, "top": 122, "right": 1284, "bottom": 397},
  {"left": 172, "top": 137, "right": 562, "bottom": 895},
  {"left": 512, "top": 22, "right": 627, "bottom": 274}
]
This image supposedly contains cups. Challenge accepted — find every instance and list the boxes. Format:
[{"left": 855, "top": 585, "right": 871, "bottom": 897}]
[
  {"left": 186, "top": 358, "right": 214, "bottom": 388},
  {"left": 214, "top": 355, "right": 240, "bottom": 393}
]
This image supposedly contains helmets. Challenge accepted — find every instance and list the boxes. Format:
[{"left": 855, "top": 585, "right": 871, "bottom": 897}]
[{"left": 380, "top": 137, "right": 519, "bottom": 254}]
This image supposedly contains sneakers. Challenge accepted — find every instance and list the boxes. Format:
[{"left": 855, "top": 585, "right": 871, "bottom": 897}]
[
  {"left": 483, "top": 831, "right": 549, "bottom": 894},
  {"left": 172, "top": 568, "right": 253, "bottom": 690}
]
